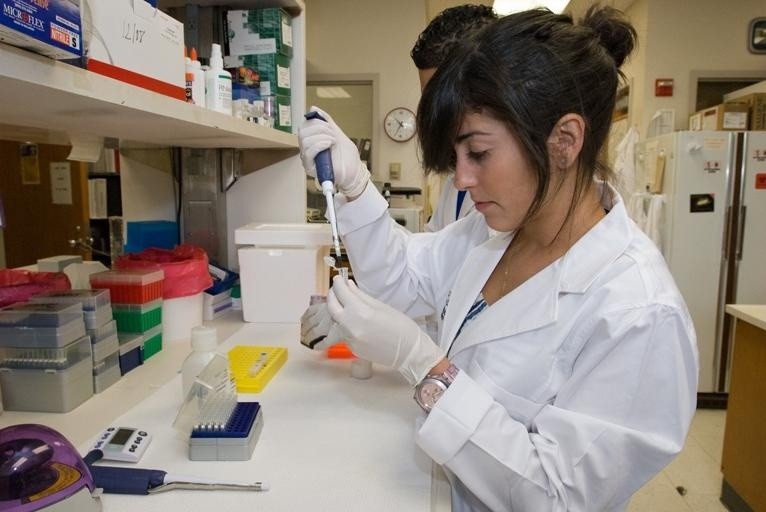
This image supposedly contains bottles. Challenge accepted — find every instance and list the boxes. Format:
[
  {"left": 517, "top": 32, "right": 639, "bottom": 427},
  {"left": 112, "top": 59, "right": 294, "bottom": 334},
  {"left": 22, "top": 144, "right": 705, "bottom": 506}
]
[
  {"left": 185, "top": 39, "right": 275, "bottom": 128},
  {"left": 191, "top": 357, "right": 238, "bottom": 430},
  {"left": 382, "top": 183, "right": 392, "bottom": 208},
  {"left": 338, "top": 267, "right": 349, "bottom": 290},
  {"left": 182, "top": 325, "right": 218, "bottom": 400}
]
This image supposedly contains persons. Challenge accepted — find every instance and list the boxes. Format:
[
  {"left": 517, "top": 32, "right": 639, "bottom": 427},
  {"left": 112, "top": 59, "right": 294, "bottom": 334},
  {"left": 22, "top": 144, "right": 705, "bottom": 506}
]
[
  {"left": 297, "top": 3, "right": 699, "bottom": 512},
  {"left": 301, "top": 4, "right": 505, "bottom": 352}
]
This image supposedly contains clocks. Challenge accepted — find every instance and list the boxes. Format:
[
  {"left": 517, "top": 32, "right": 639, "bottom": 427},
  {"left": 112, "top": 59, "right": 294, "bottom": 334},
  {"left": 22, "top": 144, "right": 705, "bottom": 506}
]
[{"left": 383, "top": 107, "right": 418, "bottom": 143}]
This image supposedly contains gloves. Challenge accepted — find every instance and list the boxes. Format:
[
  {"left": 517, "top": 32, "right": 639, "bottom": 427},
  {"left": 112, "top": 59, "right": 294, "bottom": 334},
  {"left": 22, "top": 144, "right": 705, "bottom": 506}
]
[
  {"left": 326, "top": 275, "right": 445, "bottom": 388},
  {"left": 298, "top": 106, "right": 371, "bottom": 199}
]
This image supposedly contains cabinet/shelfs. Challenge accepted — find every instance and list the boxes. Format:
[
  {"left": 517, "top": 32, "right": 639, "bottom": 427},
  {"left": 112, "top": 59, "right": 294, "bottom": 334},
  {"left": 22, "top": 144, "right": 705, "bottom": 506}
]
[{"left": 0, "top": 0, "right": 307, "bottom": 274}]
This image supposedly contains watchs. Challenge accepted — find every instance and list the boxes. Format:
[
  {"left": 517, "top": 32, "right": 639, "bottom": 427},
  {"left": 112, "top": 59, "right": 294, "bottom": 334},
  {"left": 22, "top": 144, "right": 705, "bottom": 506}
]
[{"left": 412, "top": 366, "right": 460, "bottom": 414}]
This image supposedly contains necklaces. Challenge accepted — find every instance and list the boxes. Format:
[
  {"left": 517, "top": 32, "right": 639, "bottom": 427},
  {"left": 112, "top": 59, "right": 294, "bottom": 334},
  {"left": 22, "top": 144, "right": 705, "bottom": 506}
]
[{"left": 498, "top": 238, "right": 551, "bottom": 299}]
